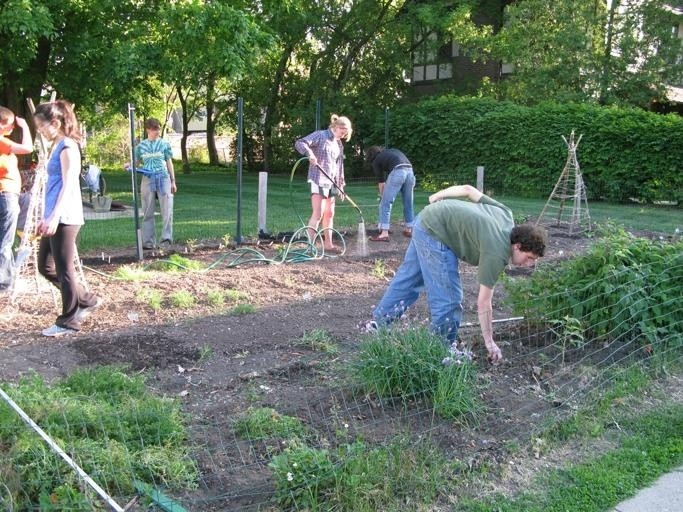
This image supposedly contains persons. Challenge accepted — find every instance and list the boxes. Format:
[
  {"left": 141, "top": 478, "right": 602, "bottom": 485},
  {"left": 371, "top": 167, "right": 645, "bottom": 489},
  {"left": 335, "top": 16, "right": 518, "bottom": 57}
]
[
  {"left": 132, "top": 117, "right": 178, "bottom": 252},
  {"left": 367, "top": 145, "right": 416, "bottom": 240},
  {"left": 294, "top": 113, "right": 354, "bottom": 256},
  {"left": 33, "top": 98, "right": 105, "bottom": 338},
  {"left": 0, "top": 104, "right": 33, "bottom": 296},
  {"left": 368, "top": 184, "right": 546, "bottom": 360}
]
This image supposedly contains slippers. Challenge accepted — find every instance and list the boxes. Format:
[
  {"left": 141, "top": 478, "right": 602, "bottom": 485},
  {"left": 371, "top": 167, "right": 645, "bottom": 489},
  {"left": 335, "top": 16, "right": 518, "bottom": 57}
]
[{"left": 325, "top": 246, "right": 342, "bottom": 253}]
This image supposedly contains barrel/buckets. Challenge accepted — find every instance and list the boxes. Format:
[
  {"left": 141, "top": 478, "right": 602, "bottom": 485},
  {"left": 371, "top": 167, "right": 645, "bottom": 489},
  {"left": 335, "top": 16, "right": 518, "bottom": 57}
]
[{"left": 91, "top": 193, "right": 113, "bottom": 213}]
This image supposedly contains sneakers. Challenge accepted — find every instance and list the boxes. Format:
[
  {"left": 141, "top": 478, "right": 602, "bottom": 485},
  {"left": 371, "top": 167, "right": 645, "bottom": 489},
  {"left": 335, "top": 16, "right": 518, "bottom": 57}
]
[
  {"left": 403, "top": 230, "right": 412, "bottom": 237},
  {"left": 370, "top": 236, "right": 390, "bottom": 241},
  {"left": 158, "top": 240, "right": 170, "bottom": 249},
  {"left": 144, "top": 243, "right": 152, "bottom": 248},
  {"left": 42, "top": 324, "right": 73, "bottom": 336},
  {"left": 75, "top": 297, "right": 103, "bottom": 320},
  {"left": 356, "top": 321, "right": 378, "bottom": 335},
  {"left": 460, "top": 349, "right": 475, "bottom": 358}
]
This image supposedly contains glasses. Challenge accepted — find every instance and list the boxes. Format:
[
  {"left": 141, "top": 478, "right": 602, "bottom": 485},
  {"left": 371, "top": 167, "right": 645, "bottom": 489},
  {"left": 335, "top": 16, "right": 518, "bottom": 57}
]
[{"left": 37, "top": 122, "right": 53, "bottom": 134}]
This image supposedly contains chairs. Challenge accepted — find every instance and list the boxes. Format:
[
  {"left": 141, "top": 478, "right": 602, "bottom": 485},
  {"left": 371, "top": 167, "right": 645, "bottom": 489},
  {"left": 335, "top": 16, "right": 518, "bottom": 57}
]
[{"left": 16, "top": 202, "right": 43, "bottom": 264}]
